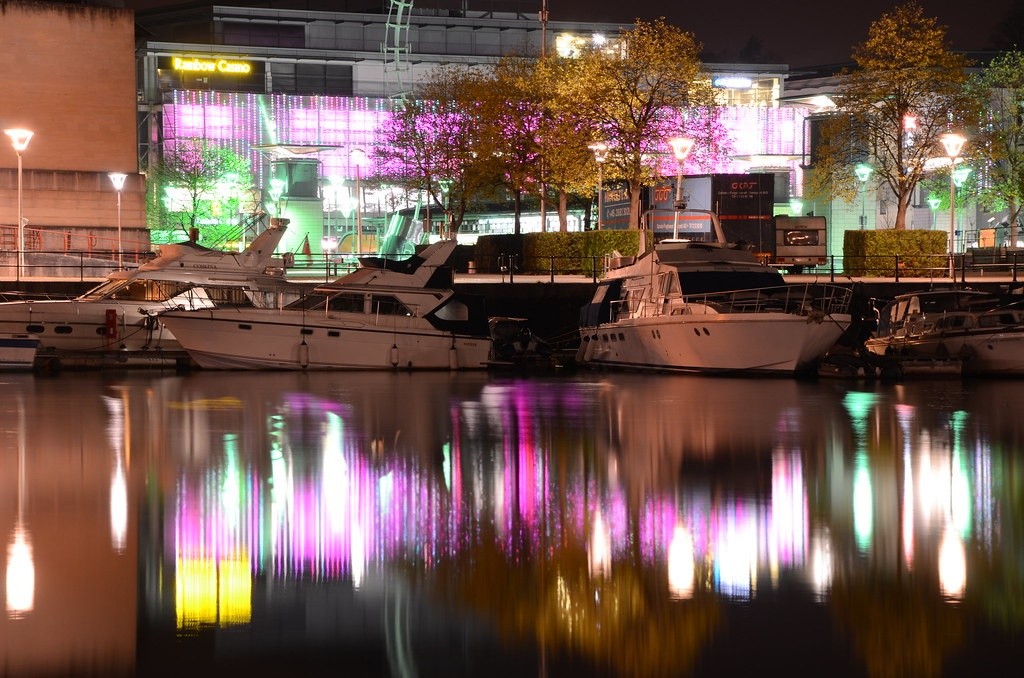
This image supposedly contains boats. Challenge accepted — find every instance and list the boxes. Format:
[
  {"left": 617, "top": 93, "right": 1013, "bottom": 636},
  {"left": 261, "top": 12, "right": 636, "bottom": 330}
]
[
  {"left": 575, "top": 208, "right": 855, "bottom": 379},
  {"left": 865, "top": 288, "right": 1024, "bottom": 376},
  {"left": 480, "top": 327, "right": 556, "bottom": 377},
  {"left": 0, "top": 218, "right": 291, "bottom": 371},
  {"left": 819, "top": 341, "right": 962, "bottom": 377},
  {"left": 156, "top": 240, "right": 491, "bottom": 371}
]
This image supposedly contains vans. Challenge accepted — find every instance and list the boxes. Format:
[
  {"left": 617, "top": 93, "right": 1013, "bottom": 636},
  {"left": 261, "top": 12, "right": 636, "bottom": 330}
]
[{"left": 775, "top": 214, "right": 828, "bottom": 274}]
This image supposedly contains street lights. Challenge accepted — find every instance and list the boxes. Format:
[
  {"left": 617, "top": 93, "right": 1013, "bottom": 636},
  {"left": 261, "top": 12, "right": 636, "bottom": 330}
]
[
  {"left": 940, "top": 134, "right": 968, "bottom": 278},
  {"left": 950, "top": 168, "right": 972, "bottom": 254},
  {"left": 589, "top": 144, "right": 616, "bottom": 230},
  {"left": 791, "top": 202, "right": 804, "bottom": 216},
  {"left": 3, "top": 128, "right": 33, "bottom": 276},
  {"left": 108, "top": 172, "right": 128, "bottom": 271},
  {"left": 270, "top": 179, "right": 286, "bottom": 217},
  {"left": 856, "top": 166, "right": 874, "bottom": 229},
  {"left": 927, "top": 199, "right": 941, "bottom": 230},
  {"left": 339, "top": 204, "right": 351, "bottom": 233},
  {"left": 269, "top": 190, "right": 283, "bottom": 257},
  {"left": 668, "top": 138, "right": 695, "bottom": 238}
]
